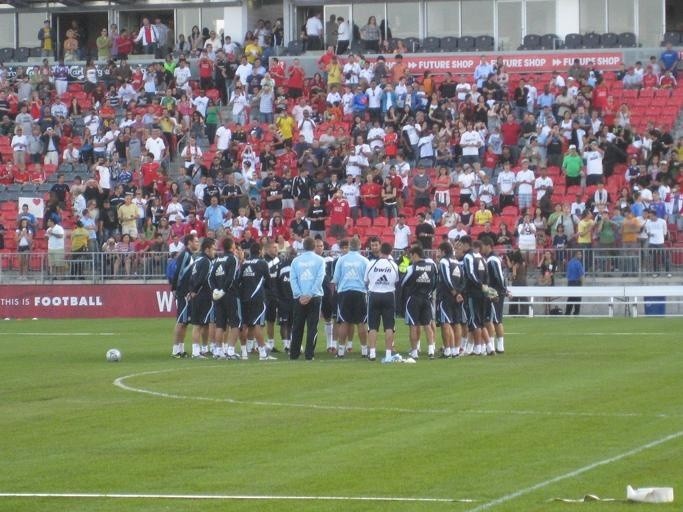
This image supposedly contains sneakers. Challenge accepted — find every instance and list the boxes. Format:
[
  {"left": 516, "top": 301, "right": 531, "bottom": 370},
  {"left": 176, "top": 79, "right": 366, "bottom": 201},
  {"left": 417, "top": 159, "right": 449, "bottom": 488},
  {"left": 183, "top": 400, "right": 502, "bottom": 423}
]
[{"left": 169, "top": 343, "right": 507, "bottom": 364}]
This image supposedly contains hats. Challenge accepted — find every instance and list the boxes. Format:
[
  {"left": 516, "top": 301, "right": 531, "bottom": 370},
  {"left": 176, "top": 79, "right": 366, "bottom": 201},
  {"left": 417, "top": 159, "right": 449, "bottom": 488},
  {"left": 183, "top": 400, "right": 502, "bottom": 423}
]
[{"left": 72, "top": 66, "right": 670, "bottom": 237}]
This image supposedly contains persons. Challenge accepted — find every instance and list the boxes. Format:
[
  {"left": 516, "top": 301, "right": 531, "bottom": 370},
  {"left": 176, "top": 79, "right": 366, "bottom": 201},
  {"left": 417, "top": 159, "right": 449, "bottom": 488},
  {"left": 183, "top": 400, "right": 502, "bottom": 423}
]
[
  {"left": 166, "top": 234, "right": 513, "bottom": 363},
  {"left": 1, "top": 12, "right": 683, "bottom": 316}
]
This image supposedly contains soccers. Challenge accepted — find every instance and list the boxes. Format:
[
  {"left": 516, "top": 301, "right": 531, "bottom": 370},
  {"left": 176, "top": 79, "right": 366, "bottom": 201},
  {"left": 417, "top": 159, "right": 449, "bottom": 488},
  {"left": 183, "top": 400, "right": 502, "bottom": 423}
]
[{"left": 106, "top": 349, "right": 121, "bottom": 362}]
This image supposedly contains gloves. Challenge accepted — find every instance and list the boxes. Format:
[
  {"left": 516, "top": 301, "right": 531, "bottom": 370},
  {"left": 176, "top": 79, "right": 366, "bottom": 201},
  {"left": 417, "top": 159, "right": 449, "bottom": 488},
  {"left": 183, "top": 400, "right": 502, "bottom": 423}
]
[{"left": 211, "top": 288, "right": 225, "bottom": 302}]
